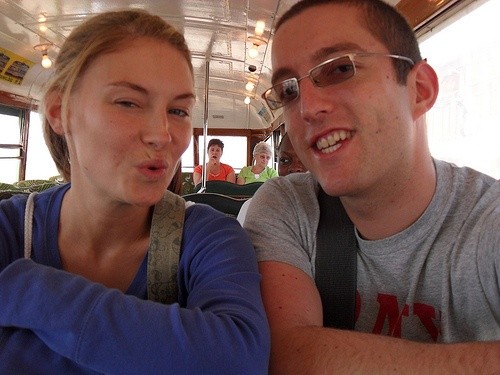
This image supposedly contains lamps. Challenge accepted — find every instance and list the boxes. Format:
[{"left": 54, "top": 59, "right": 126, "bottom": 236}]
[
  {"left": 247, "top": 36, "right": 268, "bottom": 58},
  {"left": 32, "top": 43, "right": 55, "bottom": 69}
]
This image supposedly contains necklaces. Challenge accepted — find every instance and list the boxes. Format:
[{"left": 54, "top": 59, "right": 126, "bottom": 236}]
[{"left": 209, "top": 172, "right": 221, "bottom": 177}]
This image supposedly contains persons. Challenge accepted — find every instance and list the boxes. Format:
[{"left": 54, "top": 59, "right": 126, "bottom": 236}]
[
  {"left": 237, "top": 131, "right": 309, "bottom": 229},
  {"left": 237, "top": 141, "right": 279, "bottom": 185},
  {"left": 244, "top": 0, "right": 498, "bottom": 375},
  {"left": 193, "top": 138, "right": 236, "bottom": 188},
  {"left": 0, "top": 9, "right": 274, "bottom": 375}
]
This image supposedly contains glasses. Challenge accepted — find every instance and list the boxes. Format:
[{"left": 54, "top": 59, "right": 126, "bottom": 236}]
[
  {"left": 256, "top": 155, "right": 271, "bottom": 160},
  {"left": 262, "top": 50, "right": 415, "bottom": 111}
]
